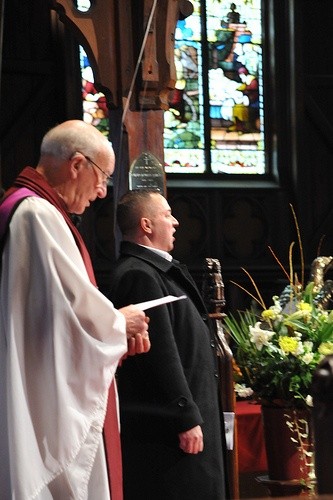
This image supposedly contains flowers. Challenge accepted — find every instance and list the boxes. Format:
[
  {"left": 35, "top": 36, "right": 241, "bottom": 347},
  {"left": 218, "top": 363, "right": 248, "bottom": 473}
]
[{"left": 221, "top": 201, "right": 333, "bottom": 411}]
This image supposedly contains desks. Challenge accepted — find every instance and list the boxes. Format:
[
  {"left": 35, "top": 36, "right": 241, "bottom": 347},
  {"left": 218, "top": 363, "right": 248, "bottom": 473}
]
[{"left": 234, "top": 399, "right": 281, "bottom": 474}]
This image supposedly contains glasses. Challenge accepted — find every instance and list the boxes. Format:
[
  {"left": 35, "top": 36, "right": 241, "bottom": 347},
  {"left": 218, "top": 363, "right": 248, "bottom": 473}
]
[{"left": 67, "top": 150, "right": 114, "bottom": 186}]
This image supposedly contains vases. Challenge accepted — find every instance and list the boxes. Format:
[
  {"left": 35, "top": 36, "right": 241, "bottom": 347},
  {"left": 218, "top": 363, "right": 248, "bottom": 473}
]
[{"left": 261, "top": 405, "right": 319, "bottom": 496}]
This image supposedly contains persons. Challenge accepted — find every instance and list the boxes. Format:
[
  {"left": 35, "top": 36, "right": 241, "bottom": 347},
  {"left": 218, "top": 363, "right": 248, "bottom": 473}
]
[
  {"left": 109, "top": 189, "right": 229, "bottom": 500},
  {"left": 1, "top": 119, "right": 152, "bottom": 499}
]
